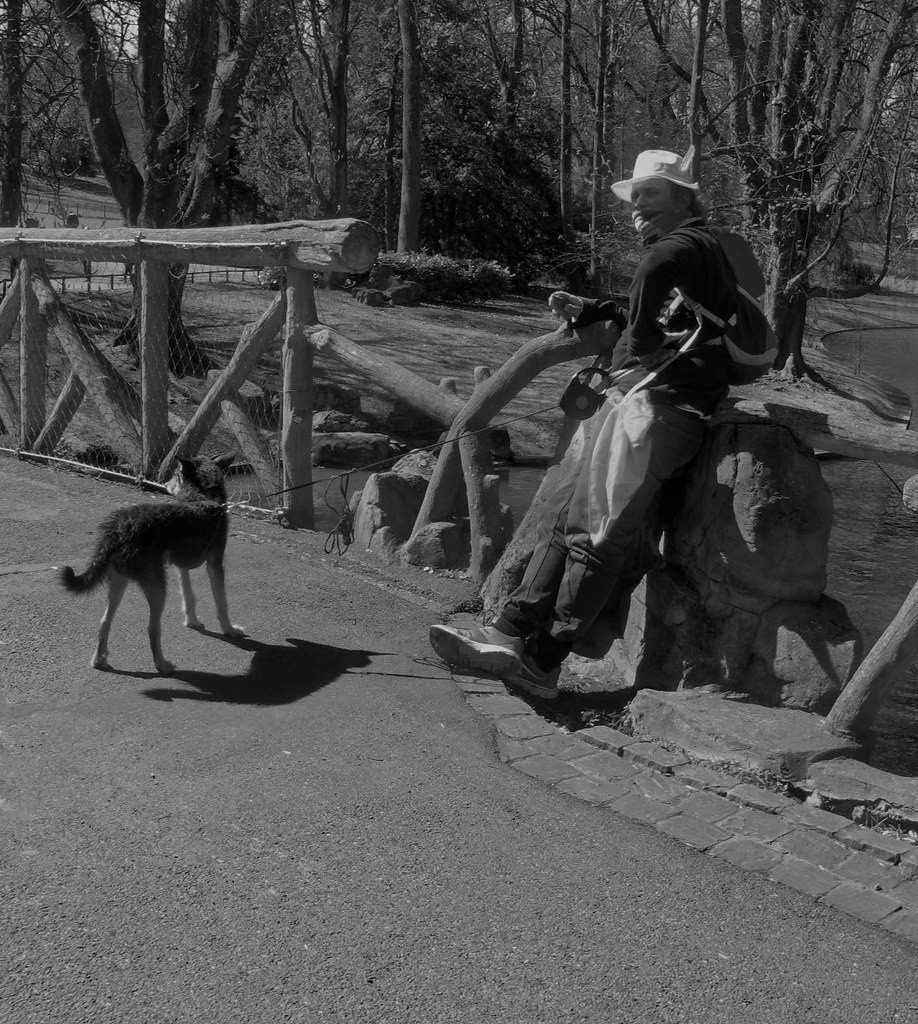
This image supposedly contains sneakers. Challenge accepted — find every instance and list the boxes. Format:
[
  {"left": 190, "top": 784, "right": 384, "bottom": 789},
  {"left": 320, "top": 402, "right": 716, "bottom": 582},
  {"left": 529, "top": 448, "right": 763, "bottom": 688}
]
[
  {"left": 429, "top": 610, "right": 525, "bottom": 677},
  {"left": 506, "top": 654, "right": 561, "bottom": 699}
]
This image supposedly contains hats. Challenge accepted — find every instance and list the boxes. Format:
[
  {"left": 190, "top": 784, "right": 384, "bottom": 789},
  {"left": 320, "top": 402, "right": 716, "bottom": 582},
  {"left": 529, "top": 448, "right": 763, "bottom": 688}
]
[{"left": 610, "top": 144, "right": 700, "bottom": 203}]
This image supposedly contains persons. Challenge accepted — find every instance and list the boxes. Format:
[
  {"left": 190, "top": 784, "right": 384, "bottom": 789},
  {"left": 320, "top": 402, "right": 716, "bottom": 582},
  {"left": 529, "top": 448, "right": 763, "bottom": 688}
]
[{"left": 429, "top": 150, "right": 761, "bottom": 699}]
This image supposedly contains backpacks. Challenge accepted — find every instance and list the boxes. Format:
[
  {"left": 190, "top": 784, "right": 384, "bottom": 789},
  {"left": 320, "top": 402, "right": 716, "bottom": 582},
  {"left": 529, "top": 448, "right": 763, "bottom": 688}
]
[{"left": 657, "top": 226, "right": 779, "bottom": 385}]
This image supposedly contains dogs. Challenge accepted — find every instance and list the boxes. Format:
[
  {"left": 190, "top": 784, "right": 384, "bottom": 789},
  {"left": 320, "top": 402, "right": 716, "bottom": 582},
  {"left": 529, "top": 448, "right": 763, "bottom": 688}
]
[{"left": 60, "top": 451, "right": 245, "bottom": 676}]
